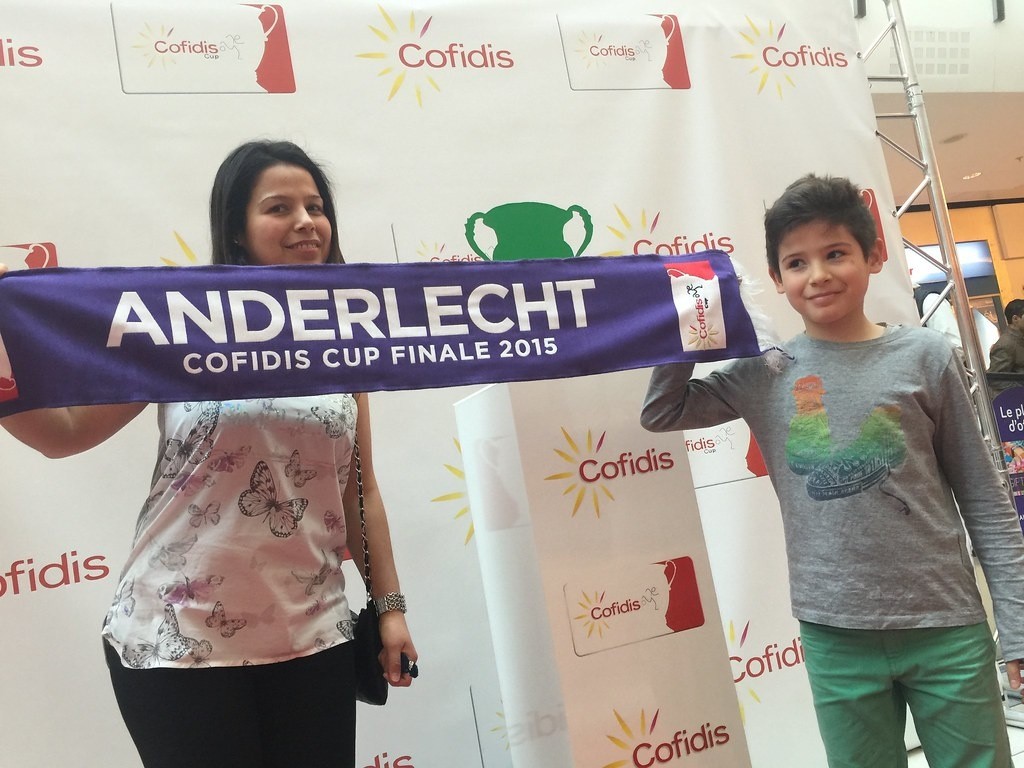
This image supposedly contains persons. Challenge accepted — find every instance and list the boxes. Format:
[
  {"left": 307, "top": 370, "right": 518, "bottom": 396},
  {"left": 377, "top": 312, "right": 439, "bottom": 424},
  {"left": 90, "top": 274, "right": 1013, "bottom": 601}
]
[
  {"left": 639, "top": 174, "right": 1024, "bottom": 768},
  {"left": 986, "top": 298, "right": 1024, "bottom": 400},
  {"left": 1, "top": 138, "right": 419, "bottom": 768}
]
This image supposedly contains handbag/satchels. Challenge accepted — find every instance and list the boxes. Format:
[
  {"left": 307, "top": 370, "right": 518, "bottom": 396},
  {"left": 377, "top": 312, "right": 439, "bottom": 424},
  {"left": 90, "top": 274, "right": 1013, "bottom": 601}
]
[{"left": 350, "top": 598, "right": 390, "bottom": 706}]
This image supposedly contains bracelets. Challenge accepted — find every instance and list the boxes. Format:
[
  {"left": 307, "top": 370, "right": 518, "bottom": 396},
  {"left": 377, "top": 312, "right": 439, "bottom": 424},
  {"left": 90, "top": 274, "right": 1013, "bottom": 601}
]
[{"left": 374, "top": 592, "right": 407, "bottom": 617}]
